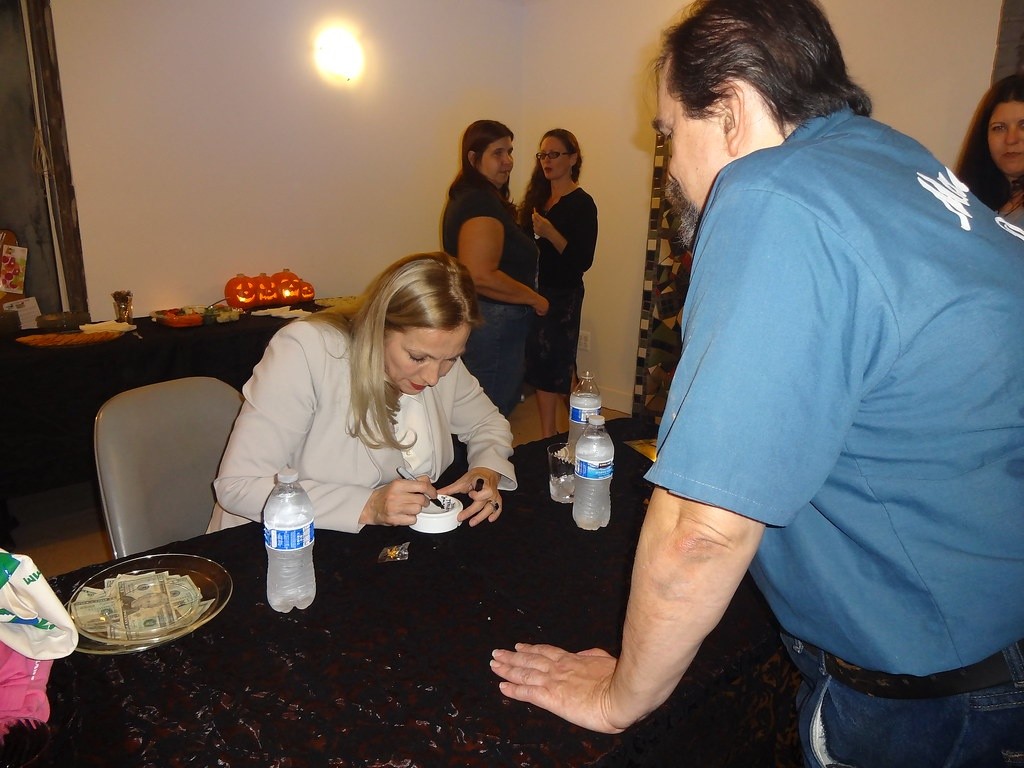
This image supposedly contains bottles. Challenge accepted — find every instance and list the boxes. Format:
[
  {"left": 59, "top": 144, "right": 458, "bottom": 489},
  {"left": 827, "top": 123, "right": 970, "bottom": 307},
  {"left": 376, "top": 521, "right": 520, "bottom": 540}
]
[
  {"left": 262, "top": 468, "right": 318, "bottom": 614},
  {"left": 567, "top": 369, "right": 603, "bottom": 466},
  {"left": 572, "top": 414, "right": 614, "bottom": 531}
]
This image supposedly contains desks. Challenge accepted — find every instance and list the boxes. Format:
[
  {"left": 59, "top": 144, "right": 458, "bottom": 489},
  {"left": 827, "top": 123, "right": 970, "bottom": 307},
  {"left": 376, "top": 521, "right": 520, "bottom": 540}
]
[
  {"left": 0, "top": 416, "right": 803, "bottom": 768},
  {"left": 0, "top": 297, "right": 343, "bottom": 499}
]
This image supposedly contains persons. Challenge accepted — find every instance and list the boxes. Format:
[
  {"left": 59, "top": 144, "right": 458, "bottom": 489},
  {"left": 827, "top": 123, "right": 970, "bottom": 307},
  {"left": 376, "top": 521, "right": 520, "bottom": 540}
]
[
  {"left": 442, "top": 118, "right": 551, "bottom": 422},
  {"left": 950, "top": 71, "right": 1024, "bottom": 230},
  {"left": 491, "top": 0, "right": 1024, "bottom": 768},
  {"left": 204, "top": 250, "right": 520, "bottom": 539},
  {"left": 512, "top": 126, "right": 600, "bottom": 443}
]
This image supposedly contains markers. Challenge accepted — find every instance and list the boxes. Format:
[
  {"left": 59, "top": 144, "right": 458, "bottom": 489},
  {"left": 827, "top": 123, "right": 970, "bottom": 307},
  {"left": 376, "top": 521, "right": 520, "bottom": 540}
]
[{"left": 396, "top": 467, "right": 444, "bottom": 509}]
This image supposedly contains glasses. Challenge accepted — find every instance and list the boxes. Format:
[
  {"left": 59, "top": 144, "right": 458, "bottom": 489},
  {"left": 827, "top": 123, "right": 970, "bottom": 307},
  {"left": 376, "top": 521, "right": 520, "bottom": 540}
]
[{"left": 536, "top": 151, "right": 569, "bottom": 161}]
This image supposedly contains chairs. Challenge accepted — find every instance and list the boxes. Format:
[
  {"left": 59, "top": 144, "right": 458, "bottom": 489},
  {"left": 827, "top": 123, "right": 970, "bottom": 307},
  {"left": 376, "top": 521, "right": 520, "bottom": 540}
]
[{"left": 93, "top": 376, "right": 245, "bottom": 560}]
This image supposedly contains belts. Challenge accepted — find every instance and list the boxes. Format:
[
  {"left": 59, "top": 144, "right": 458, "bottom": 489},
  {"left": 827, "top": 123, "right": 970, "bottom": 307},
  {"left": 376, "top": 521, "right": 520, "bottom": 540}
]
[{"left": 794, "top": 630, "right": 1024, "bottom": 700}]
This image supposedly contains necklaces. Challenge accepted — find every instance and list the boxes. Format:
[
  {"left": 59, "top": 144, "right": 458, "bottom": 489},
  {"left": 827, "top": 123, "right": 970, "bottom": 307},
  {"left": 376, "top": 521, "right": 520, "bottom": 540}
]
[{"left": 543, "top": 185, "right": 569, "bottom": 216}]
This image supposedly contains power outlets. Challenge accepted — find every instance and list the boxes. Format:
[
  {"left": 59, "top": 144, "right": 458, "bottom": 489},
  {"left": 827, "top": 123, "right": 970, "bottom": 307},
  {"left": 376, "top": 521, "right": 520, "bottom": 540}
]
[{"left": 577, "top": 330, "right": 592, "bottom": 351}]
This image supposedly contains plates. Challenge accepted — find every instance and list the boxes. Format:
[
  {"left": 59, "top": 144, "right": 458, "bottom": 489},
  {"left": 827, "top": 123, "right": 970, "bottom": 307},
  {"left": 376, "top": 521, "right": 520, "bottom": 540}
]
[{"left": 64, "top": 553, "right": 234, "bottom": 656}]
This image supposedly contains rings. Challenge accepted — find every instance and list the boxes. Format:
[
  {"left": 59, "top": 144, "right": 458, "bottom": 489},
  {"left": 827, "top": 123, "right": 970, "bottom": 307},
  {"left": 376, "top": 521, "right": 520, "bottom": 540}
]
[{"left": 489, "top": 500, "right": 500, "bottom": 510}]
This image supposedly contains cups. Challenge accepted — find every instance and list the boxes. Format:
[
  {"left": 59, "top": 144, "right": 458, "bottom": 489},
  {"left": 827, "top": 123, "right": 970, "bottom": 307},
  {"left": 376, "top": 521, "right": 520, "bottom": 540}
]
[
  {"left": 545, "top": 442, "right": 575, "bottom": 503},
  {"left": 112, "top": 301, "right": 135, "bottom": 324}
]
